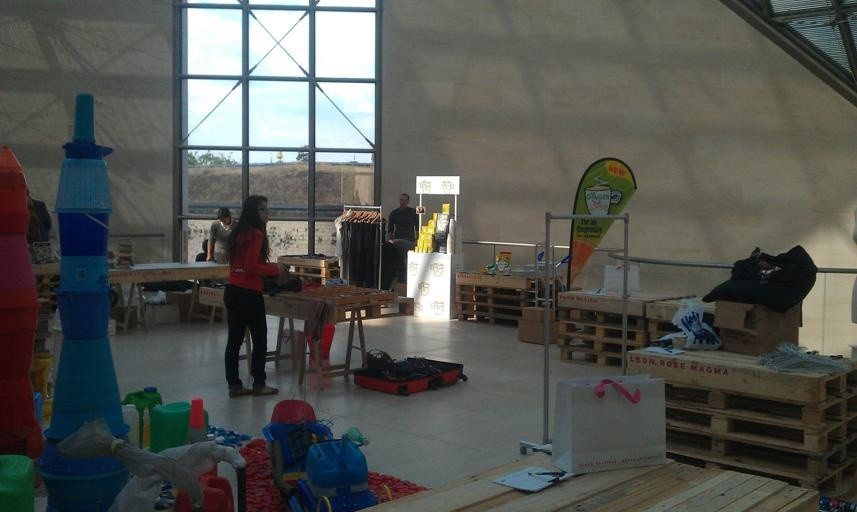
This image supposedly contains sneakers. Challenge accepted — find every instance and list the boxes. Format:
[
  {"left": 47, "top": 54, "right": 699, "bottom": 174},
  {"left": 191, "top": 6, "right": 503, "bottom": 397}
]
[
  {"left": 229, "top": 388, "right": 252, "bottom": 398},
  {"left": 253, "top": 386, "right": 278, "bottom": 396}
]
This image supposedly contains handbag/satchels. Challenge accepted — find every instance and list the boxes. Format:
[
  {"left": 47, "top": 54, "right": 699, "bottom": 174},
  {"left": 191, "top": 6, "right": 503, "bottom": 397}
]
[
  {"left": 548, "top": 373, "right": 666, "bottom": 472},
  {"left": 703, "top": 245, "right": 819, "bottom": 314},
  {"left": 604, "top": 262, "right": 640, "bottom": 290}
]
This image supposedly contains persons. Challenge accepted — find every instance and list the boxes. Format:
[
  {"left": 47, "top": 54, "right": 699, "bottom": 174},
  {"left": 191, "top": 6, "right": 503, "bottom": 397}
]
[
  {"left": 381, "top": 238, "right": 416, "bottom": 289},
  {"left": 224, "top": 195, "right": 286, "bottom": 398},
  {"left": 207, "top": 208, "right": 240, "bottom": 263},
  {"left": 388, "top": 193, "right": 418, "bottom": 241}
]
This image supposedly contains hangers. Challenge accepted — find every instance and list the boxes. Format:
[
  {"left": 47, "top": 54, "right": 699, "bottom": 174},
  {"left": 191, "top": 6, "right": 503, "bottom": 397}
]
[{"left": 340, "top": 208, "right": 387, "bottom": 223}]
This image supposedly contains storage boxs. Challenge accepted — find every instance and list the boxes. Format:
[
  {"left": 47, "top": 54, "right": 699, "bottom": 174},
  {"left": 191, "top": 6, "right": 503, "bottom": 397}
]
[{"left": 712, "top": 299, "right": 801, "bottom": 356}]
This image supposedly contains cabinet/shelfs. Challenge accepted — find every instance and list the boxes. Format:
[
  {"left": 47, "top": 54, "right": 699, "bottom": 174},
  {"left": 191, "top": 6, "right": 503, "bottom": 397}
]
[
  {"left": 646, "top": 296, "right": 716, "bottom": 345},
  {"left": 344, "top": 454, "right": 820, "bottom": 512},
  {"left": 558, "top": 286, "right": 698, "bottom": 369},
  {"left": 276, "top": 254, "right": 340, "bottom": 285},
  {"left": 407, "top": 175, "right": 464, "bottom": 319},
  {"left": 456, "top": 270, "right": 554, "bottom": 326},
  {"left": 626, "top": 345, "right": 856, "bottom": 494}
]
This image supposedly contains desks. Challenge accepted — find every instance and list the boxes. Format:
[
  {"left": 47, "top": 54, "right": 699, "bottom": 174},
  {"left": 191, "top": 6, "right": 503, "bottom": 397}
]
[
  {"left": 201, "top": 287, "right": 398, "bottom": 385},
  {"left": 108, "top": 262, "right": 230, "bottom": 335}
]
